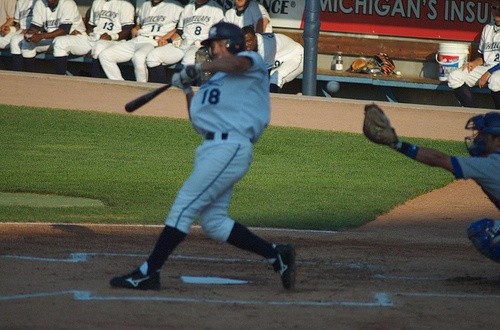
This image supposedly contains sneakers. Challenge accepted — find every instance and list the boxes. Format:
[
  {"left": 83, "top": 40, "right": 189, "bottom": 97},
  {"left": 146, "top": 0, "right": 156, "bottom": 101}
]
[
  {"left": 110, "top": 269, "right": 160, "bottom": 291},
  {"left": 270, "top": 242, "right": 295, "bottom": 289}
]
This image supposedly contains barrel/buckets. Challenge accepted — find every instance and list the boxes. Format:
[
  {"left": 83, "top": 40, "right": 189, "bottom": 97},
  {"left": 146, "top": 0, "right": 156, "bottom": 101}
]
[{"left": 435, "top": 42, "right": 469, "bottom": 83}]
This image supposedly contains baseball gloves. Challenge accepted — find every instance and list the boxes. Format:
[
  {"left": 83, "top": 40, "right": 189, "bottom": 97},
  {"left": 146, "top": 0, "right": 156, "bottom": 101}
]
[{"left": 363, "top": 104, "right": 398, "bottom": 144}]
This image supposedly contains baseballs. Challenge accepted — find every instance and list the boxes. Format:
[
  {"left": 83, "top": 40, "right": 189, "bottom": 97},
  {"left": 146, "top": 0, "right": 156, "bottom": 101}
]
[{"left": 327, "top": 80, "right": 340, "bottom": 93}]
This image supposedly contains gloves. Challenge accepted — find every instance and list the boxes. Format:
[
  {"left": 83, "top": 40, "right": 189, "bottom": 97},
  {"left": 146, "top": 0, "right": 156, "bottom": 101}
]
[
  {"left": 179, "top": 62, "right": 202, "bottom": 83},
  {"left": 170, "top": 73, "right": 191, "bottom": 94}
]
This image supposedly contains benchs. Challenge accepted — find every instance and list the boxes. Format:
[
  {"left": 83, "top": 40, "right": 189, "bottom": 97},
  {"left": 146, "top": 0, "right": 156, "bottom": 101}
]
[{"left": 2, "top": 26, "right": 498, "bottom": 98}]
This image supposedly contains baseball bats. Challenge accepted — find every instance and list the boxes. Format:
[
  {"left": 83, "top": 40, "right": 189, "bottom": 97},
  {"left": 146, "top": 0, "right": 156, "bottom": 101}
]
[{"left": 125, "top": 75, "right": 184, "bottom": 112}]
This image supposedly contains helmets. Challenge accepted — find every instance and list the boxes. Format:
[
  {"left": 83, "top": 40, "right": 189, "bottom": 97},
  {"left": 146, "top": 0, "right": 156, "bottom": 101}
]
[{"left": 463, "top": 111, "right": 500, "bottom": 156}]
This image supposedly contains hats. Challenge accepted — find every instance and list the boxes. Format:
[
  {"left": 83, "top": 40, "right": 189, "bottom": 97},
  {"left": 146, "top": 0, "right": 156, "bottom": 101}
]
[{"left": 199, "top": 23, "right": 244, "bottom": 54}]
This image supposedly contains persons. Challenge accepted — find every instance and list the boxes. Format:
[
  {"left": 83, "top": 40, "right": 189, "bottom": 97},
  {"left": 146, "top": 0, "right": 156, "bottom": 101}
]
[
  {"left": 361, "top": 102, "right": 499, "bottom": 268},
  {"left": 52, "top": 0, "right": 135, "bottom": 76},
  {"left": 444, "top": 0, "right": 500, "bottom": 109},
  {"left": 224, "top": 0, "right": 274, "bottom": 35},
  {"left": 108, "top": 19, "right": 299, "bottom": 293},
  {"left": 0, "top": 0, "right": 18, "bottom": 35},
  {"left": 146, "top": 0, "right": 228, "bottom": 89},
  {"left": 240, "top": 24, "right": 306, "bottom": 94},
  {"left": 20, "top": 0, "right": 89, "bottom": 77},
  {"left": 1, "top": 0, "right": 36, "bottom": 72},
  {"left": 98, "top": 0, "right": 187, "bottom": 83}
]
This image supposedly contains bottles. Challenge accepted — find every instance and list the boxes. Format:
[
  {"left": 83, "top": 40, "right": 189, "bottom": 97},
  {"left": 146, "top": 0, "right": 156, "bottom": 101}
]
[{"left": 336, "top": 51, "right": 344, "bottom": 71}]
